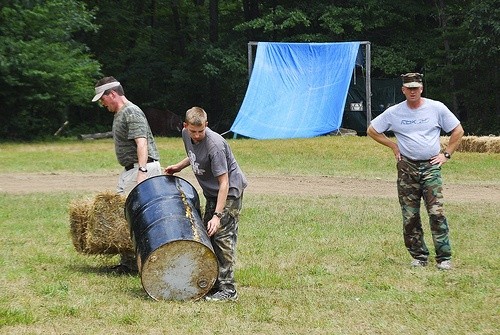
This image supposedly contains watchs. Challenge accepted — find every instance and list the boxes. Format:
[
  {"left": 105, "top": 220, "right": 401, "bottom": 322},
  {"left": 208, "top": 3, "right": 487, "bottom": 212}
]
[
  {"left": 441, "top": 150, "right": 450, "bottom": 159},
  {"left": 213, "top": 211, "right": 222, "bottom": 218},
  {"left": 138, "top": 166, "right": 148, "bottom": 172}
]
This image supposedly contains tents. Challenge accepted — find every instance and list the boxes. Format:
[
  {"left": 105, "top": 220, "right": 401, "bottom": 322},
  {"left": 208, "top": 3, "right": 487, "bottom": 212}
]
[{"left": 229, "top": 40, "right": 371, "bottom": 140}]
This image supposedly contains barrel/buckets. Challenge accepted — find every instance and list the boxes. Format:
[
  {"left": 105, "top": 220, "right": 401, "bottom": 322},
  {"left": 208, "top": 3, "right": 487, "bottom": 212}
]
[{"left": 124, "top": 174, "right": 220, "bottom": 304}]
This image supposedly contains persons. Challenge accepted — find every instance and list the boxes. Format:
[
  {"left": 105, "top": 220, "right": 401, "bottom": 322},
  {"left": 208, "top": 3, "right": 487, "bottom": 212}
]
[
  {"left": 92, "top": 76, "right": 161, "bottom": 275},
  {"left": 368, "top": 72, "right": 464, "bottom": 269},
  {"left": 165, "top": 106, "right": 248, "bottom": 302}
]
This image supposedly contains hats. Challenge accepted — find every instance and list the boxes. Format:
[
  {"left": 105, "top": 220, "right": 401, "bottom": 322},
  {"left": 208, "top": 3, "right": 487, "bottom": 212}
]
[
  {"left": 92, "top": 82, "right": 120, "bottom": 102},
  {"left": 402, "top": 73, "right": 423, "bottom": 87}
]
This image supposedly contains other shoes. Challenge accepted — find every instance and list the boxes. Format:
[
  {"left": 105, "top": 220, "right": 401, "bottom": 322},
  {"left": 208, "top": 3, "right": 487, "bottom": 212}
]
[
  {"left": 436, "top": 260, "right": 452, "bottom": 270},
  {"left": 414, "top": 260, "right": 427, "bottom": 267},
  {"left": 107, "top": 265, "right": 138, "bottom": 275}
]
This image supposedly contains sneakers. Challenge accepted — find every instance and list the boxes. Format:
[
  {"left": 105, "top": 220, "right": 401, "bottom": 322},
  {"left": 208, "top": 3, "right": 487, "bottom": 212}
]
[{"left": 205, "top": 289, "right": 238, "bottom": 302}]
